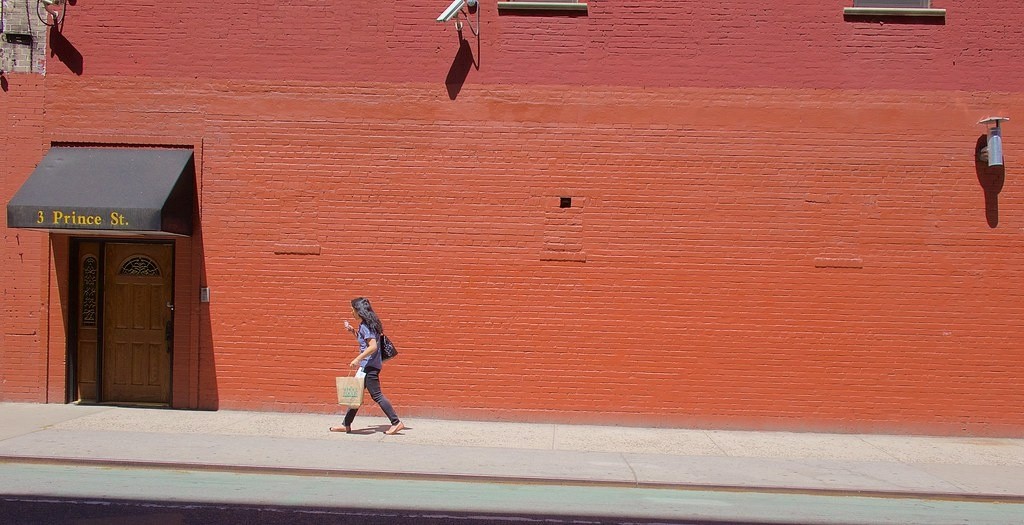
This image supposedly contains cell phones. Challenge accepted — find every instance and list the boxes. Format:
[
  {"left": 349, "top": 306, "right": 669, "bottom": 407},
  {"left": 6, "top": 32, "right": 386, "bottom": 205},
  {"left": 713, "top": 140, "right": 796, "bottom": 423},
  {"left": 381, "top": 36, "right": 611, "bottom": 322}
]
[{"left": 344, "top": 320, "right": 349, "bottom": 329}]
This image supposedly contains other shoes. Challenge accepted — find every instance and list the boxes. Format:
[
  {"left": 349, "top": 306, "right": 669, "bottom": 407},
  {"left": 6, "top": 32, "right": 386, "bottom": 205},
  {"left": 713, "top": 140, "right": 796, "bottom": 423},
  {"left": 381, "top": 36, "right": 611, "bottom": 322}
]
[
  {"left": 384, "top": 421, "right": 405, "bottom": 435},
  {"left": 330, "top": 426, "right": 351, "bottom": 432}
]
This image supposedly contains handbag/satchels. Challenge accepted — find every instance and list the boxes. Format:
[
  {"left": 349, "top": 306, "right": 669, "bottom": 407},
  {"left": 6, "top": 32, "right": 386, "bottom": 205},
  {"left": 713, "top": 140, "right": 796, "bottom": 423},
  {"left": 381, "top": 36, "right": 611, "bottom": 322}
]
[
  {"left": 380, "top": 332, "right": 398, "bottom": 362},
  {"left": 336, "top": 367, "right": 365, "bottom": 409}
]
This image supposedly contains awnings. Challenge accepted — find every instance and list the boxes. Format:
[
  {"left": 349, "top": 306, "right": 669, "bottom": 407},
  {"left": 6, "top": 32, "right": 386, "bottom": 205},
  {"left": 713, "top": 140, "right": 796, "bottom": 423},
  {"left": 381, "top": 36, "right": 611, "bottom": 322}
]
[{"left": 7, "top": 148, "right": 194, "bottom": 237}]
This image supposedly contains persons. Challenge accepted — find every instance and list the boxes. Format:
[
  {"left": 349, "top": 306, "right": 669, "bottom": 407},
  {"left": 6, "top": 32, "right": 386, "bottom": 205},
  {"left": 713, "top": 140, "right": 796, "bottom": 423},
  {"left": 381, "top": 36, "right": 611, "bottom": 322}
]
[{"left": 330, "top": 298, "right": 404, "bottom": 434}]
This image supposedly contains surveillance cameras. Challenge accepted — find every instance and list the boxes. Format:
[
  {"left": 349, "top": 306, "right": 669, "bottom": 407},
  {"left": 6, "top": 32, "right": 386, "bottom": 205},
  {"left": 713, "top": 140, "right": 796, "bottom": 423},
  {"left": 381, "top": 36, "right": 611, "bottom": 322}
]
[
  {"left": 435, "top": 0, "right": 466, "bottom": 23},
  {"left": 987, "top": 126, "right": 1003, "bottom": 167}
]
